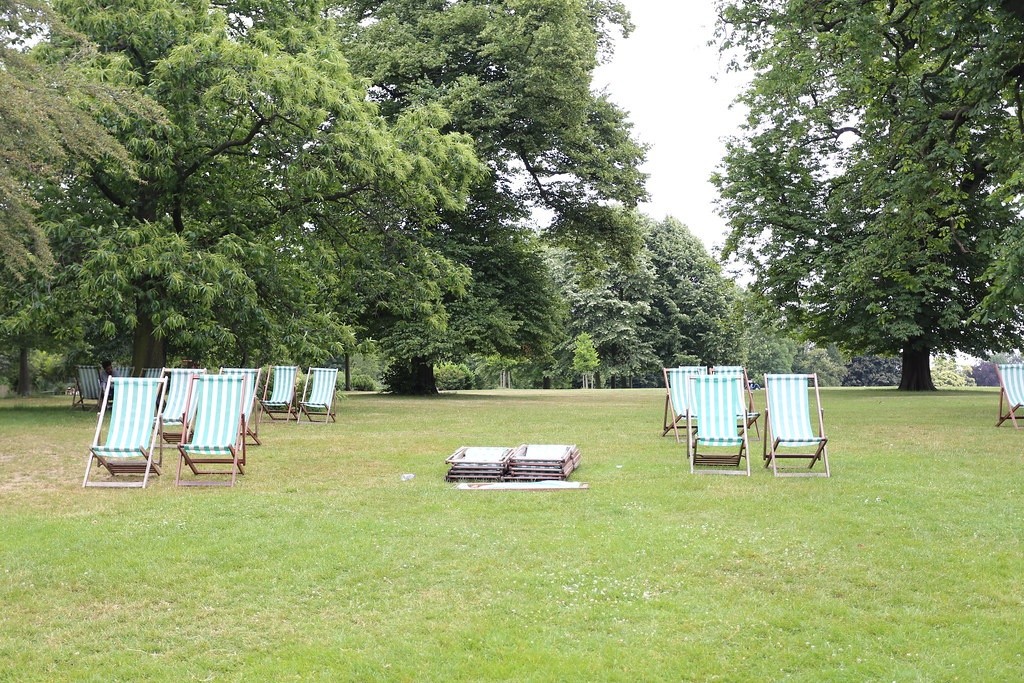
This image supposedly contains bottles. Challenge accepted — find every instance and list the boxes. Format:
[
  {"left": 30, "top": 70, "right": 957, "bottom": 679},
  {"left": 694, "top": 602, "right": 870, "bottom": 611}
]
[{"left": 401, "top": 473, "right": 416, "bottom": 482}]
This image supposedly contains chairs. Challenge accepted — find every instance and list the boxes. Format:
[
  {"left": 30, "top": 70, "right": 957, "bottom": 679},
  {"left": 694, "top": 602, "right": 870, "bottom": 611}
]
[
  {"left": 152, "top": 367, "right": 207, "bottom": 449},
  {"left": 296, "top": 366, "right": 338, "bottom": 423},
  {"left": 71, "top": 364, "right": 101, "bottom": 412},
  {"left": 83, "top": 374, "right": 168, "bottom": 488},
  {"left": 138, "top": 367, "right": 162, "bottom": 379},
  {"left": 219, "top": 367, "right": 262, "bottom": 445},
  {"left": 764, "top": 373, "right": 830, "bottom": 477},
  {"left": 663, "top": 367, "right": 697, "bottom": 443},
  {"left": 679, "top": 366, "right": 708, "bottom": 376},
  {"left": 444, "top": 443, "right": 583, "bottom": 483},
  {"left": 687, "top": 373, "right": 751, "bottom": 477},
  {"left": 107, "top": 366, "right": 135, "bottom": 404},
  {"left": 176, "top": 372, "right": 246, "bottom": 487},
  {"left": 995, "top": 364, "right": 1024, "bottom": 430},
  {"left": 258, "top": 365, "right": 299, "bottom": 423},
  {"left": 710, "top": 366, "right": 762, "bottom": 441}
]
